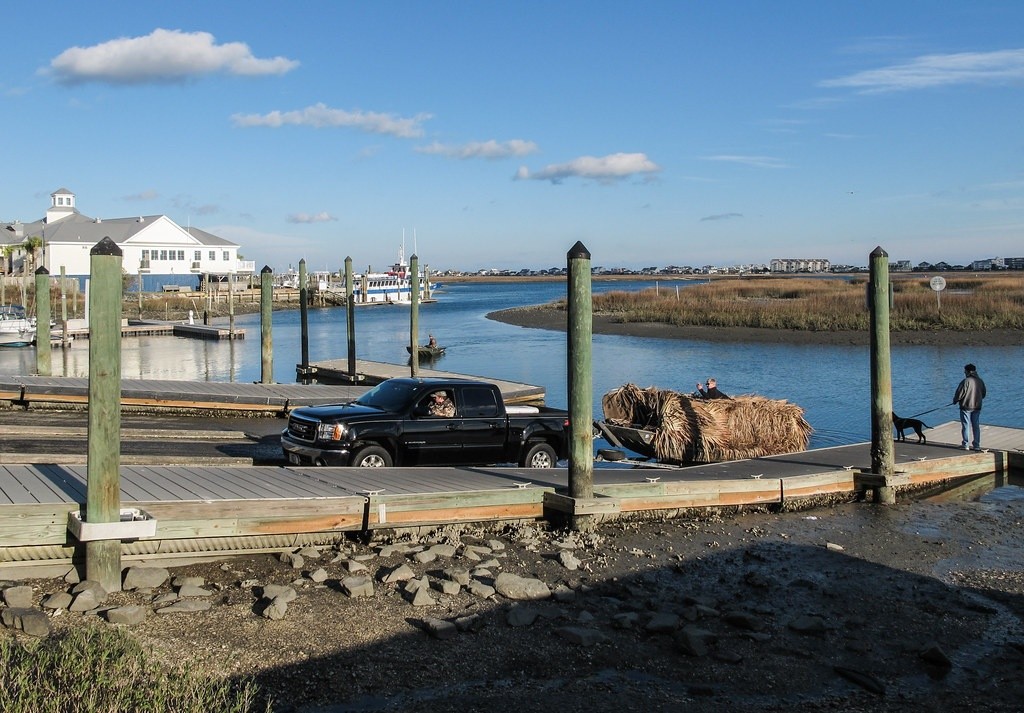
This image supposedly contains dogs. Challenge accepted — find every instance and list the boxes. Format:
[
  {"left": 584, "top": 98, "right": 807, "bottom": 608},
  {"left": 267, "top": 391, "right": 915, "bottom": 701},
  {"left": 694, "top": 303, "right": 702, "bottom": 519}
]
[{"left": 892, "top": 411, "right": 932, "bottom": 444}]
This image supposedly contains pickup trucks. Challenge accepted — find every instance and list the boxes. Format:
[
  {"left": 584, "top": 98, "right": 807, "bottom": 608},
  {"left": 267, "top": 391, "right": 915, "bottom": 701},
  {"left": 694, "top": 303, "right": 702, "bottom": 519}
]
[{"left": 280, "top": 377, "right": 569, "bottom": 467}]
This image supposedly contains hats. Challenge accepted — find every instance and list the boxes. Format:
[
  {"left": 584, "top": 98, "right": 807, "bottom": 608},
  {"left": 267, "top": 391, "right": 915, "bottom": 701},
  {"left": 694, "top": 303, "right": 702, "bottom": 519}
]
[
  {"left": 964, "top": 364, "right": 976, "bottom": 371},
  {"left": 431, "top": 391, "right": 447, "bottom": 397}
]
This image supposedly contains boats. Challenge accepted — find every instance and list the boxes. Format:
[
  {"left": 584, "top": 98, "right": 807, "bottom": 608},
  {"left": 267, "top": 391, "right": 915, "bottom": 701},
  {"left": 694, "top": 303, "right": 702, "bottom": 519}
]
[
  {"left": 0, "top": 305, "right": 36, "bottom": 347},
  {"left": 405, "top": 344, "right": 447, "bottom": 359},
  {"left": 593, "top": 383, "right": 815, "bottom": 459},
  {"left": 331, "top": 228, "right": 441, "bottom": 299}
]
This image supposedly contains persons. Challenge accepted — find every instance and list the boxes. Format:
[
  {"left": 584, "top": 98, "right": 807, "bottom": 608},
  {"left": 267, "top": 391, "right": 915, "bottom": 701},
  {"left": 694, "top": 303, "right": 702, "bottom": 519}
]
[
  {"left": 952, "top": 364, "right": 986, "bottom": 451},
  {"left": 696, "top": 378, "right": 732, "bottom": 401},
  {"left": 428, "top": 334, "right": 436, "bottom": 348},
  {"left": 426, "top": 390, "right": 455, "bottom": 418}
]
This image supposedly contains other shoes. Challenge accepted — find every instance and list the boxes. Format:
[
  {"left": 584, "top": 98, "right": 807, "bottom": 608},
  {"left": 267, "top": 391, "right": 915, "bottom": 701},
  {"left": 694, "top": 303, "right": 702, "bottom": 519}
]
[
  {"left": 960, "top": 447, "right": 969, "bottom": 450},
  {"left": 970, "top": 447, "right": 980, "bottom": 450}
]
